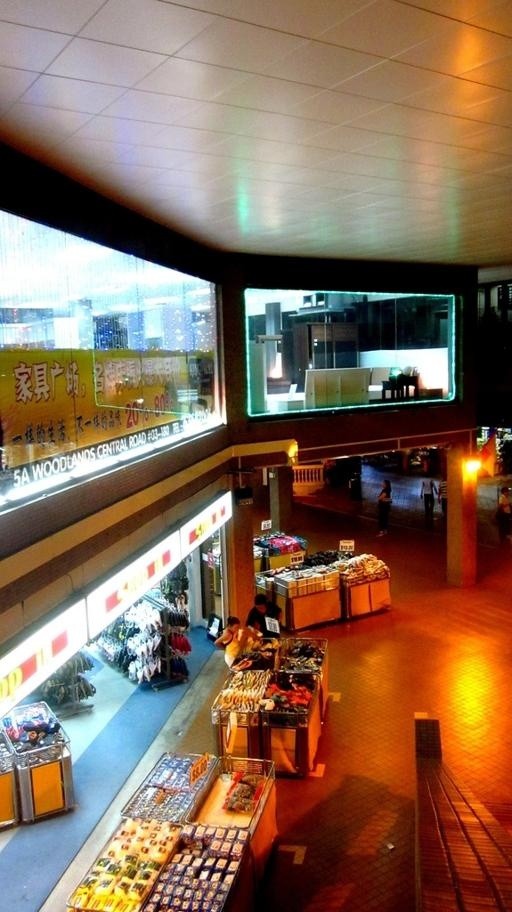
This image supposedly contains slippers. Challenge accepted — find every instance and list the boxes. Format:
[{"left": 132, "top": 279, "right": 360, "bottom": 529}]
[
  {"left": 41, "top": 652, "right": 96, "bottom": 703},
  {"left": 95, "top": 600, "right": 162, "bottom": 684}
]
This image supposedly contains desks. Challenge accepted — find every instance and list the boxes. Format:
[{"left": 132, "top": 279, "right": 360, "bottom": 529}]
[
  {"left": 418, "top": 388, "right": 443, "bottom": 400},
  {"left": 381, "top": 381, "right": 409, "bottom": 399}
]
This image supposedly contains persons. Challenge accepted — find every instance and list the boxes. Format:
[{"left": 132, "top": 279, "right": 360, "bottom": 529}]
[
  {"left": 214, "top": 616, "right": 241, "bottom": 669},
  {"left": 245, "top": 593, "right": 283, "bottom": 642},
  {"left": 374, "top": 479, "right": 393, "bottom": 537},
  {"left": 419, "top": 475, "right": 438, "bottom": 518},
  {"left": 438, "top": 473, "right": 447, "bottom": 521},
  {"left": 497, "top": 486, "right": 512, "bottom": 539}
]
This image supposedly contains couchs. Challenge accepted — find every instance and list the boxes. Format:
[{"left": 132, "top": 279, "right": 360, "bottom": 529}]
[{"left": 358, "top": 367, "right": 415, "bottom": 400}]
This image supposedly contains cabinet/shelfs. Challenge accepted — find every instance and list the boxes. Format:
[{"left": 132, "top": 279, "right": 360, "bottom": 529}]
[{"left": 293, "top": 322, "right": 360, "bottom": 392}]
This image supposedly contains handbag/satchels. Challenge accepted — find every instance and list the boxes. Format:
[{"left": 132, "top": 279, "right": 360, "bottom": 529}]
[
  {"left": 214, "top": 636, "right": 225, "bottom": 649},
  {"left": 383, "top": 498, "right": 392, "bottom": 503}
]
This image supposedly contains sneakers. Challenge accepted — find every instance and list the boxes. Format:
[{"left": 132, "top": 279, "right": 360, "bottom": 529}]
[
  {"left": 343, "top": 569, "right": 357, "bottom": 579},
  {"left": 265, "top": 699, "right": 275, "bottom": 711},
  {"left": 377, "top": 533, "right": 384, "bottom": 537}
]
